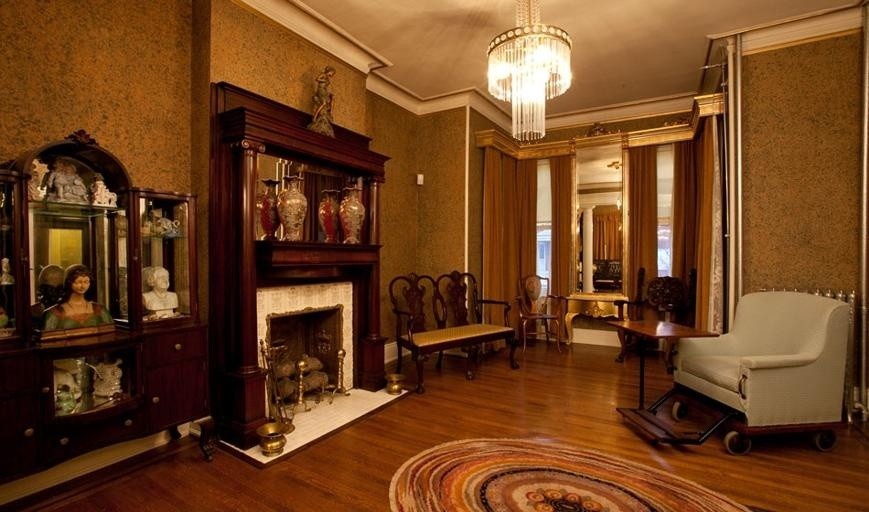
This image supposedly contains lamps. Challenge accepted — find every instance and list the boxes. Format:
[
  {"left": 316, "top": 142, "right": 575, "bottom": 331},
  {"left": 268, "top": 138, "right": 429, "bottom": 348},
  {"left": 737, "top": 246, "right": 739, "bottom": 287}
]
[{"left": 485, "top": 1, "right": 574, "bottom": 147}]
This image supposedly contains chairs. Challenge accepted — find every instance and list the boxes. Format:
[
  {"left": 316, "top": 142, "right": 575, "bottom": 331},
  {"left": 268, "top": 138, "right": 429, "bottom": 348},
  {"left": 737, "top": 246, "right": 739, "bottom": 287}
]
[
  {"left": 514, "top": 272, "right": 572, "bottom": 362},
  {"left": 576, "top": 256, "right": 622, "bottom": 290}
]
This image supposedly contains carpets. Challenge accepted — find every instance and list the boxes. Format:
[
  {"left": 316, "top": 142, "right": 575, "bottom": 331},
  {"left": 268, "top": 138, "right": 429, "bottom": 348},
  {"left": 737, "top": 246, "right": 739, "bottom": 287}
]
[{"left": 388, "top": 432, "right": 755, "bottom": 511}]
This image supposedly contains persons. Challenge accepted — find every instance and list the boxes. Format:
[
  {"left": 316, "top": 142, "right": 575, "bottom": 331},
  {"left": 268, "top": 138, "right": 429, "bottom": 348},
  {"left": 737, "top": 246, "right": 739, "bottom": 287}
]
[
  {"left": 44, "top": 262, "right": 113, "bottom": 330},
  {"left": 142, "top": 265, "right": 181, "bottom": 311},
  {"left": 46, "top": 160, "right": 87, "bottom": 203},
  {"left": 313, "top": 64, "right": 336, "bottom": 121}
]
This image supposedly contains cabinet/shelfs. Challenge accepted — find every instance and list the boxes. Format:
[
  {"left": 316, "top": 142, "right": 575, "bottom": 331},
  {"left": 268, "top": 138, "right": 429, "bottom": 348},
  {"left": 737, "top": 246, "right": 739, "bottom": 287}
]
[
  {"left": 210, "top": 79, "right": 392, "bottom": 454},
  {"left": 0, "top": 130, "right": 219, "bottom": 493}
]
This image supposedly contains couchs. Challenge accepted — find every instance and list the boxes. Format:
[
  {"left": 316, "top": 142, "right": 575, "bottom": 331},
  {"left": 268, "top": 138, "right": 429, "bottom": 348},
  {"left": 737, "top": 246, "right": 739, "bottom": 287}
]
[{"left": 614, "top": 289, "right": 855, "bottom": 457}]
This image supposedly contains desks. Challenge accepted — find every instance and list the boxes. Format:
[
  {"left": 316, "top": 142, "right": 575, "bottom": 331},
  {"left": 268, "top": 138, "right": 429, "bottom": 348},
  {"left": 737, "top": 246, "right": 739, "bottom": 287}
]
[{"left": 604, "top": 318, "right": 721, "bottom": 374}]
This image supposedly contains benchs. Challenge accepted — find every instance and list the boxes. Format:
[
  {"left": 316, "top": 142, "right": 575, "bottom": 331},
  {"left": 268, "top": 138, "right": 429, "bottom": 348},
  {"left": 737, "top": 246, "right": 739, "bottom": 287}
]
[{"left": 388, "top": 267, "right": 521, "bottom": 395}]
